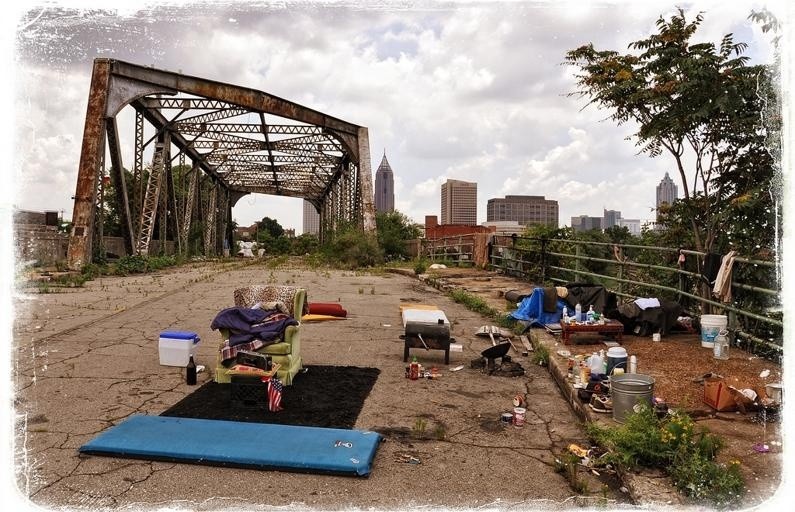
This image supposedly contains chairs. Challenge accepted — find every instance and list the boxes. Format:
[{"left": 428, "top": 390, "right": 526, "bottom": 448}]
[{"left": 217, "top": 285, "right": 305, "bottom": 387}]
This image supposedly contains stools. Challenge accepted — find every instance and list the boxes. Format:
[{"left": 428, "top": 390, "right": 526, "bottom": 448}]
[
  {"left": 399, "top": 302, "right": 446, "bottom": 326},
  {"left": 400, "top": 320, "right": 450, "bottom": 366},
  {"left": 156, "top": 330, "right": 198, "bottom": 368}
]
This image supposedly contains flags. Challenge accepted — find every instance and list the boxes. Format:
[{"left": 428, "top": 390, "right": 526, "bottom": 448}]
[{"left": 268, "top": 379, "right": 284, "bottom": 412}]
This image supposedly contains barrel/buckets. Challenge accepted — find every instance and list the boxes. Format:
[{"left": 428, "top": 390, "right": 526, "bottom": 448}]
[
  {"left": 699, "top": 314, "right": 729, "bottom": 347},
  {"left": 712, "top": 329, "right": 730, "bottom": 360},
  {"left": 608, "top": 361, "right": 654, "bottom": 425}
]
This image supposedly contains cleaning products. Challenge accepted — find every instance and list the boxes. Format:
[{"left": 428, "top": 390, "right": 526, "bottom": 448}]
[{"left": 586, "top": 304, "right": 595, "bottom": 321}]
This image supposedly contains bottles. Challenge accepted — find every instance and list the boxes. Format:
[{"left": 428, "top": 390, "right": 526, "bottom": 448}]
[
  {"left": 567, "top": 353, "right": 591, "bottom": 388},
  {"left": 186, "top": 354, "right": 198, "bottom": 386},
  {"left": 562, "top": 305, "right": 568, "bottom": 321},
  {"left": 575, "top": 303, "right": 582, "bottom": 322},
  {"left": 405, "top": 354, "right": 438, "bottom": 381}
]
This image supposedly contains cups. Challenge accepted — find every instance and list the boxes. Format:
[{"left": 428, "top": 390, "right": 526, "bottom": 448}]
[{"left": 511, "top": 408, "right": 527, "bottom": 430}]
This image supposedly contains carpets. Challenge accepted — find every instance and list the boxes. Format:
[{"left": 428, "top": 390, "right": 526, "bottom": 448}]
[{"left": 162, "top": 367, "right": 380, "bottom": 426}]
